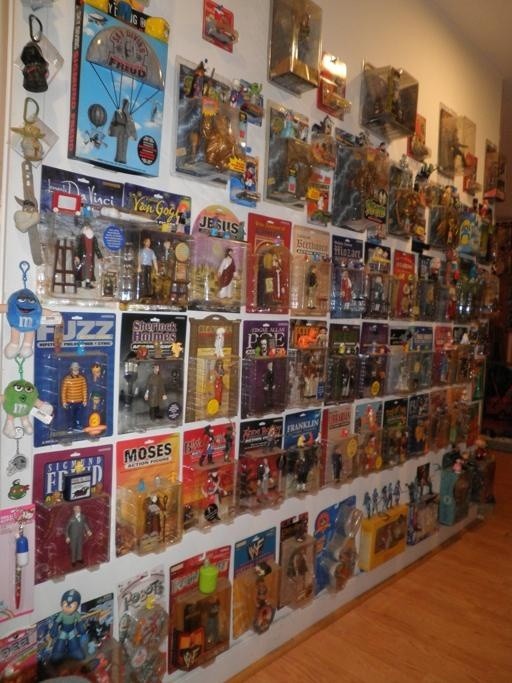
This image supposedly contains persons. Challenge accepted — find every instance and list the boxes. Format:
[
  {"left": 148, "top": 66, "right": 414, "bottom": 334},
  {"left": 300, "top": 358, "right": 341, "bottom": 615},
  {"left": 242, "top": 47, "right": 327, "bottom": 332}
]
[
  {"left": 0, "top": 0, "right": 497, "bottom": 682},
  {"left": 2, "top": 0, "right": 497, "bottom": 682}
]
